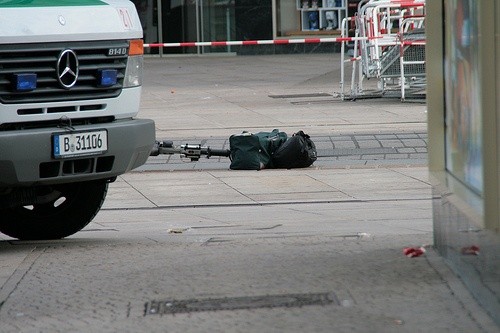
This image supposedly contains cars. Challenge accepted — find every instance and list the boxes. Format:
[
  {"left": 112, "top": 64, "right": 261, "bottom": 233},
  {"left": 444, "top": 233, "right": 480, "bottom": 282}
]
[{"left": 0, "top": 0, "right": 156, "bottom": 240}]
